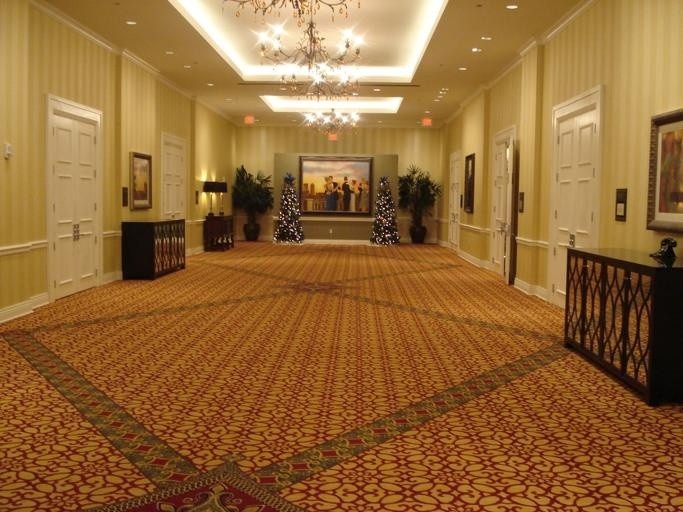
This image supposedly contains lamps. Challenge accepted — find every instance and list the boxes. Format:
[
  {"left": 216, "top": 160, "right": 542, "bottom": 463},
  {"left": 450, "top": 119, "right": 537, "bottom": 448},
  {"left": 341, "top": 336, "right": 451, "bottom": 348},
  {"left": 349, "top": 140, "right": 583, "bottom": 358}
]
[
  {"left": 219, "top": 182, "right": 227, "bottom": 216},
  {"left": 254, "top": 0, "right": 365, "bottom": 70},
  {"left": 299, "top": 106, "right": 359, "bottom": 136},
  {"left": 220, "top": 0, "right": 364, "bottom": 26},
  {"left": 280, "top": 69, "right": 360, "bottom": 99},
  {"left": 202, "top": 181, "right": 222, "bottom": 216}
]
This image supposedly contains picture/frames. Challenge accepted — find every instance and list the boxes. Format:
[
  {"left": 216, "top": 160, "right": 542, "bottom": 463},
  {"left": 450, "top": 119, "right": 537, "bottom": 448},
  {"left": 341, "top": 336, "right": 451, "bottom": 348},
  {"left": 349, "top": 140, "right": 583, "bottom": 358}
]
[
  {"left": 129, "top": 151, "right": 152, "bottom": 209},
  {"left": 463, "top": 154, "right": 475, "bottom": 213},
  {"left": 299, "top": 156, "right": 374, "bottom": 217},
  {"left": 646, "top": 106, "right": 683, "bottom": 232}
]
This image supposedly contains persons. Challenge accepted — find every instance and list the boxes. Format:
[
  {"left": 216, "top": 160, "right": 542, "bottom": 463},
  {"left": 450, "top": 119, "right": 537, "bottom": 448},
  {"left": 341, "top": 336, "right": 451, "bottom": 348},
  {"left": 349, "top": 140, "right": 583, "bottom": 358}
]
[{"left": 324, "top": 175, "right": 369, "bottom": 212}]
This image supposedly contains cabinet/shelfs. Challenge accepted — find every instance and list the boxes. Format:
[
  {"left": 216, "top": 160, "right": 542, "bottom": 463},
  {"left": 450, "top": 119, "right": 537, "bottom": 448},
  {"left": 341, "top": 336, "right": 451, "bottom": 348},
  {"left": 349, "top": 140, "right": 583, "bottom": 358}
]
[
  {"left": 565, "top": 248, "right": 683, "bottom": 406},
  {"left": 203, "top": 216, "right": 233, "bottom": 250},
  {"left": 120, "top": 218, "right": 185, "bottom": 281}
]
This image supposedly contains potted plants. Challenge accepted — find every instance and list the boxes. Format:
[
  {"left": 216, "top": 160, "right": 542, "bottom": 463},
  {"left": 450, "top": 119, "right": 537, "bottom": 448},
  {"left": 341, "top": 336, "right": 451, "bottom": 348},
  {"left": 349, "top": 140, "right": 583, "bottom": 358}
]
[
  {"left": 231, "top": 164, "right": 275, "bottom": 240},
  {"left": 397, "top": 159, "right": 444, "bottom": 243}
]
